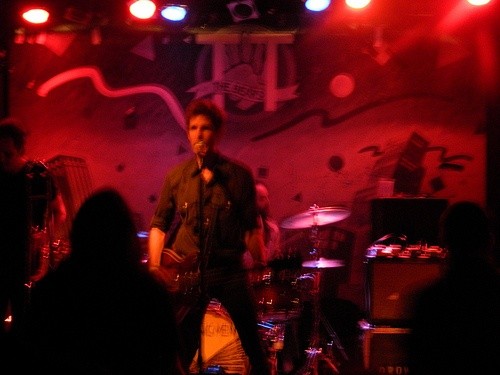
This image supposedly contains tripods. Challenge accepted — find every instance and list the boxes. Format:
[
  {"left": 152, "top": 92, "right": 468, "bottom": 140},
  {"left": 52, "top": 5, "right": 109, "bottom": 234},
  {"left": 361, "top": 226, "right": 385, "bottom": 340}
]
[{"left": 298, "top": 216, "right": 343, "bottom": 375}]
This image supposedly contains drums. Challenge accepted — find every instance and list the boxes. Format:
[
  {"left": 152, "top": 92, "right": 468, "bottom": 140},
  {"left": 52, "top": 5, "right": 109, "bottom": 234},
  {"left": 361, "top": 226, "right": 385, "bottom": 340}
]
[
  {"left": 256, "top": 322, "right": 285, "bottom": 354},
  {"left": 251, "top": 273, "right": 303, "bottom": 321}
]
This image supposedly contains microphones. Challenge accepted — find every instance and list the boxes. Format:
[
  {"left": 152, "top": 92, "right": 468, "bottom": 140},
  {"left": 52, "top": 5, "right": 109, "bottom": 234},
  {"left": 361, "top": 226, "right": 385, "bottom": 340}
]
[{"left": 194, "top": 142, "right": 205, "bottom": 164}]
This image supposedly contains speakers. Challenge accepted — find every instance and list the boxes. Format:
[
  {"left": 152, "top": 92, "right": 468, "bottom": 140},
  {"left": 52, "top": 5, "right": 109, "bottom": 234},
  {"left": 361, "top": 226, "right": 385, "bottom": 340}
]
[{"left": 366, "top": 256, "right": 446, "bottom": 325}]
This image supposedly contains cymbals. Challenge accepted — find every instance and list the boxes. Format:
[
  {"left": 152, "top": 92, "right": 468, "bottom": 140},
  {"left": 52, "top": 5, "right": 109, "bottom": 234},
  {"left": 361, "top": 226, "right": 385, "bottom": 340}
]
[
  {"left": 278, "top": 207, "right": 348, "bottom": 231},
  {"left": 301, "top": 259, "right": 344, "bottom": 268}
]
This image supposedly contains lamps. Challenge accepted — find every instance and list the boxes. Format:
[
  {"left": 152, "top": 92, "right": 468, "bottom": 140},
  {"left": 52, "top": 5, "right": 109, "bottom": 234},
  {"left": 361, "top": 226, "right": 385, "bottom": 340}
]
[
  {"left": 227, "top": 0, "right": 262, "bottom": 23},
  {"left": 159, "top": 0, "right": 193, "bottom": 25}
]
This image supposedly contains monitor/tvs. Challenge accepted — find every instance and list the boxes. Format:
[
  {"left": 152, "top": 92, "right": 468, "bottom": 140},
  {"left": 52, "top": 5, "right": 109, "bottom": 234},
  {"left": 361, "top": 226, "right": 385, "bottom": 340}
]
[{"left": 369, "top": 197, "right": 449, "bottom": 248}]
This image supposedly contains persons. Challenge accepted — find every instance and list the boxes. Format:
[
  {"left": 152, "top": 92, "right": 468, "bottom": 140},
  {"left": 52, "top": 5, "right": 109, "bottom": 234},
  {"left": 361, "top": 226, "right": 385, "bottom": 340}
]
[
  {"left": 244, "top": 183, "right": 281, "bottom": 321},
  {"left": 0, "top": 123, "right": 185, "bottom": 375},
  {"left": 149, "top": 104, "right": 272, "bottom": 375},
  {"left": 397, "top": 200, "right": 500, "bottom": 375}
]
